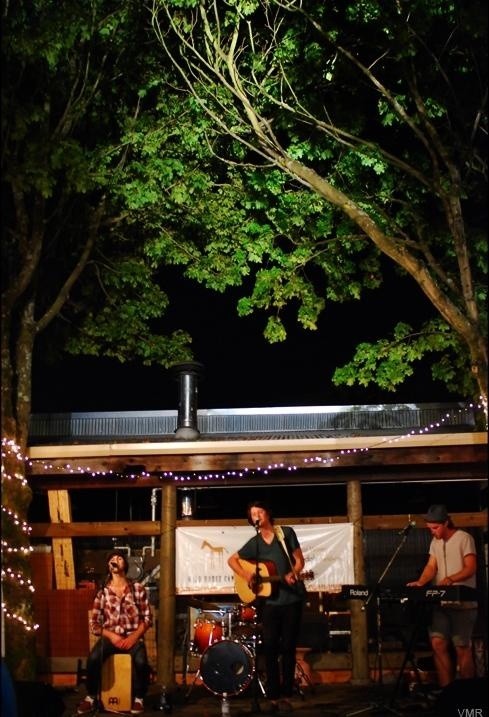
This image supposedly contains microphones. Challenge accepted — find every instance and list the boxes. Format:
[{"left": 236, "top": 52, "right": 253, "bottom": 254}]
[
  {"left": 110, "top": 561, "right": 118, "bottom": 568},
  {"left": 255, "top": 518, "right": 259, "bottom": 531},
  {"left": 401, "top": 520, "right": 416, "bottom": 535}
]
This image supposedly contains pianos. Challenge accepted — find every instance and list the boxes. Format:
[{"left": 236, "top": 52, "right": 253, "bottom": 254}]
[{"left": 342, "top": 584, "right": 485, "bottom": 601}]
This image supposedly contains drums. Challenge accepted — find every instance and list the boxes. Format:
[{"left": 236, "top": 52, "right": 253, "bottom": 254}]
[
  {"left": 191, "top": 611, "right": 223, "bottom": 656},
  {"left": 226, "top": 604, "right": 264, "bottom": 639},
  {"left": 198, "top": 639, "right": 256, "bottom": 699}
]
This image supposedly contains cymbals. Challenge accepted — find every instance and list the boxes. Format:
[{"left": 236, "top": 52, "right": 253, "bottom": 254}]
[{"left": 168, "top": 594, "right": 220, "bottom": 611}]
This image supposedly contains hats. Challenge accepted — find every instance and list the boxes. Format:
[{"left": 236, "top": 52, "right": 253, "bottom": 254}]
[
  {"left": 107, "top": 548, "right": 130, "bottom": 574},
  {"left": 423, "top": 503, "right": 450, "bottom": 524}
]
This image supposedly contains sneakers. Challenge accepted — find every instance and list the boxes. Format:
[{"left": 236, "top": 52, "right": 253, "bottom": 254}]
[
  {"left": 427, "top": 689, "right": 443, "bottom": 700},
  {"left": 77, "top": 695, "right": 95, "bottom": 714},
  {"left": 266, "top": 703, "right": 277, "bottom": 714},
  {"left": 131, "top": 697, "right": 144, "bottom": 714}
]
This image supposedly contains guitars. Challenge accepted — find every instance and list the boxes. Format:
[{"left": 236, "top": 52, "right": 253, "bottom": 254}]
[{"left": 234, "top": 556, "right": 314, "bottom": 606}]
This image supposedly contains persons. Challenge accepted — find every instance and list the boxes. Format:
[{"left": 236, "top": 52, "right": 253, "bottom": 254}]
[
  {"left": 78, "top": 549, "right": 153, "bottom": 713},
  {"left": 229, "top": 502, "right": 308, "bottom": 711},
  {"left": 1, "top": 656, "right": 20, "bottom": 714},
  {"left": 406, "top": 506, "right": 480, "bottom": 700}
]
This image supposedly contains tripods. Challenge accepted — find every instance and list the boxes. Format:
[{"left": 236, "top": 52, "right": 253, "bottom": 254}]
[
  {"left": 180, "top": 619, "right": 317, "bottom": 702},
  {"left": 345, "top": 596, "right": 406, "bottom": 717},
  {"left": 74, "top": 568, "right": 129, "bottom": 717}
]
